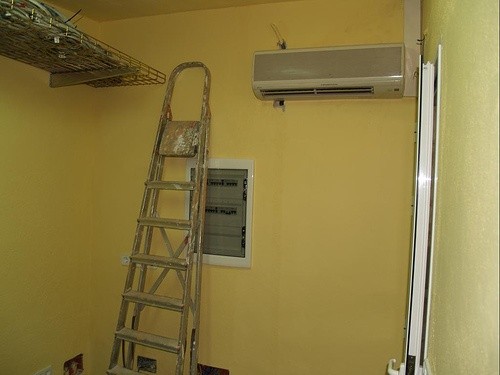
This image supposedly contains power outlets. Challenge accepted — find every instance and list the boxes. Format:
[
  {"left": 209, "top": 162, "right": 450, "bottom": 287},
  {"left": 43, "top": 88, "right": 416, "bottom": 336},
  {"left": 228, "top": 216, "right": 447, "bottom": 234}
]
[{"left": 33, "top": 364, "right": 53, "bottom": 375}]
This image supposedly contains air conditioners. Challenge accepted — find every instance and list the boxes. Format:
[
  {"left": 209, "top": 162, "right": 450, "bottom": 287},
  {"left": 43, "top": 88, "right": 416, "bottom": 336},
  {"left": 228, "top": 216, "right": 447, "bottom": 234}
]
[{"left": 252, "top": 42, "right": 406, "bottom": 103}]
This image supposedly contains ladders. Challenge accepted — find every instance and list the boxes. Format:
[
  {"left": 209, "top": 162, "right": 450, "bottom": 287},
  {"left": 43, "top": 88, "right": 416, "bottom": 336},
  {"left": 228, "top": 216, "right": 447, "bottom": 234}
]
[{"left": 105, "top": 60, "right": 212, "bottom": 375}]
